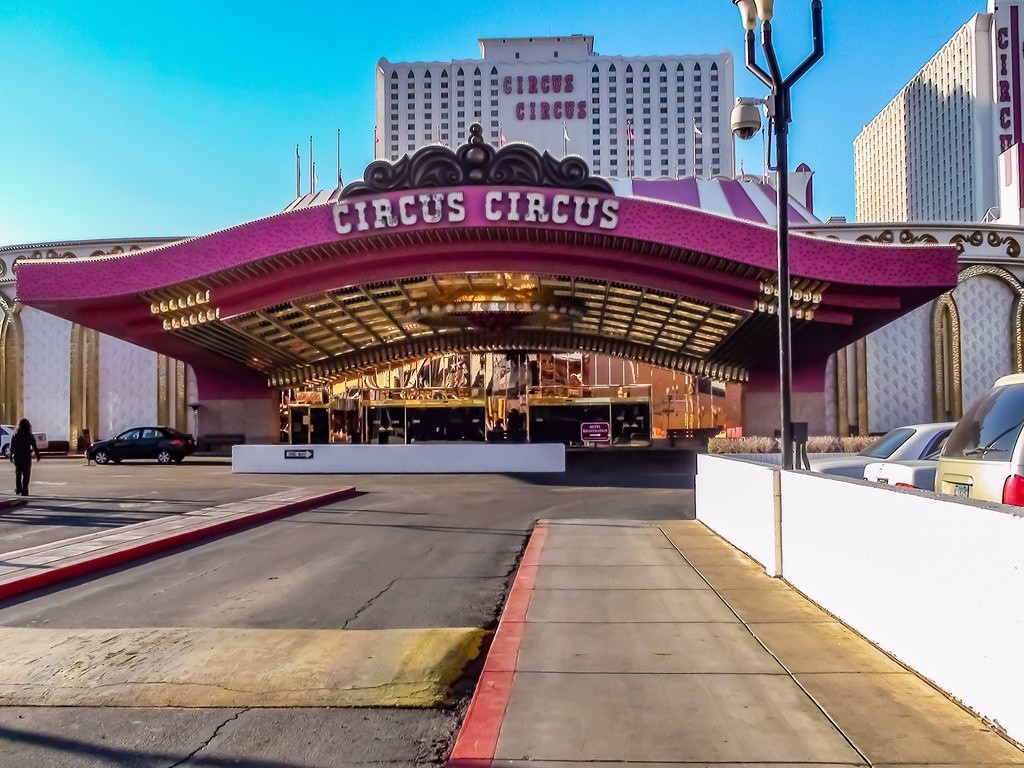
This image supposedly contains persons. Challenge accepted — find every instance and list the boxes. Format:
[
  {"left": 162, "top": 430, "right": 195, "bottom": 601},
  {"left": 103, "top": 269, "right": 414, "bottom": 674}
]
[{"left": 9, "top": 418, "right": 41, "bottom": 496}]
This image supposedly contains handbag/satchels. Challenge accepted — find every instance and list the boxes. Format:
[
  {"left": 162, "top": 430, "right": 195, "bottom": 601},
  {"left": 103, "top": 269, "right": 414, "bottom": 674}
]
[{"left": 521, "top": 431, "right": 527, "bottom": 440}]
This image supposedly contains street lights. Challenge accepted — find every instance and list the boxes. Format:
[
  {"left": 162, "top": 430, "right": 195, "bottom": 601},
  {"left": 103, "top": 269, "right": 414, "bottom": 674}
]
[{"left": 734, "top": 0, "right": 826, "bottom": 471}]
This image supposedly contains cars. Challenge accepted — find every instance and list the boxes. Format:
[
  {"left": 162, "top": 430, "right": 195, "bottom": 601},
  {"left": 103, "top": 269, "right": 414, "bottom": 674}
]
[
  {"left": 0, "top": 425, "right": 49, "bottom": 458},
  {"left": 862, "top": 446, "right": 943, "bottom": 494},
  {"left": 799, "top": 421, "right": 958, "bottom": 481},
  {"left": 85, "top": 425, "right": 197, "bottom": 464}
]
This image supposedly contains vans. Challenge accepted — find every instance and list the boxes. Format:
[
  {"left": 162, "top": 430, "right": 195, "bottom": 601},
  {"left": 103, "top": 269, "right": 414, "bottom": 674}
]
[{"left": 935, "top": 373, "right": 1024, "bottom": 508}]
